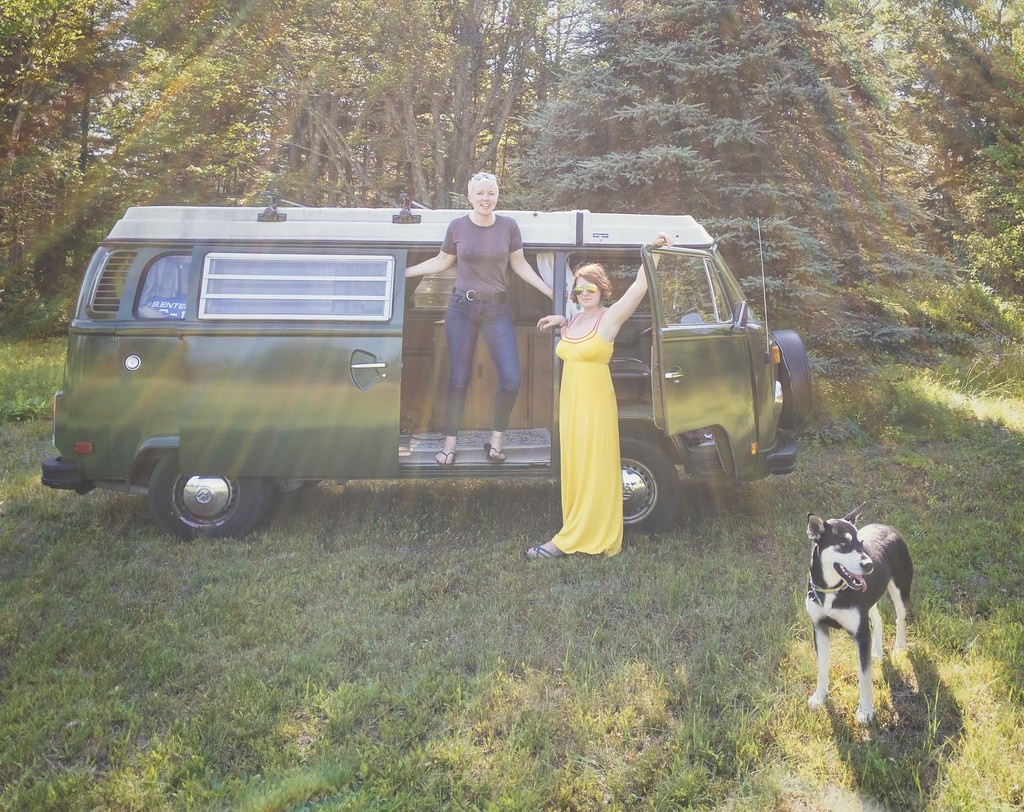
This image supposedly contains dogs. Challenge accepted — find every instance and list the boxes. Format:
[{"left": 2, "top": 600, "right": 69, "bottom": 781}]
[{"left": 806, "top": 501, "right": 914, "bottom": 726}]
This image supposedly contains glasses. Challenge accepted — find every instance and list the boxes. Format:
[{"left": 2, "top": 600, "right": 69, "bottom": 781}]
[
  {"left": 470, "top": 174, "right": 496, "bottom": 183},
  {"left": 573, "top": 284, "right": 598, "bottom": 295}
]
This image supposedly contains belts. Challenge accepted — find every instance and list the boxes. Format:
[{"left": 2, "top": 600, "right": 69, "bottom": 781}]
[{"left": 452, "top": 289, "right": 507, "bottom": 302}]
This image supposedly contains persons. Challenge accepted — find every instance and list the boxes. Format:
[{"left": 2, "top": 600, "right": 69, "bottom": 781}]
[
  {"left": 523, "top": 232, "right": 673, "bottom": 560},
  {"left": 404, "top": 172, "right": 553, "bottom": 467}
]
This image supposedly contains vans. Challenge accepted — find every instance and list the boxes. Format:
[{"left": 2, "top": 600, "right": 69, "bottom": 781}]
[{"left": 40, "top": 201, "right": 814, "bottom": 543}]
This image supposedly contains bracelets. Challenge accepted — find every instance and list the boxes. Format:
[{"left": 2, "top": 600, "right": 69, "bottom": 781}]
[{"left": 556, "top": 320, "right": 568, "bottom": 329}]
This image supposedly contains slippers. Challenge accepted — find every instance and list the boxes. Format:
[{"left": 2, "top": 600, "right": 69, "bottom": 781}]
[
  {"left": 483, "top": 443, "right": 505, "bottom": 462},
  {"left": 524, "top": 541, "right": 571, "bottom": 561},
  {"left": 435, "top": 452, "right": 456, "bottom": 468}
]
